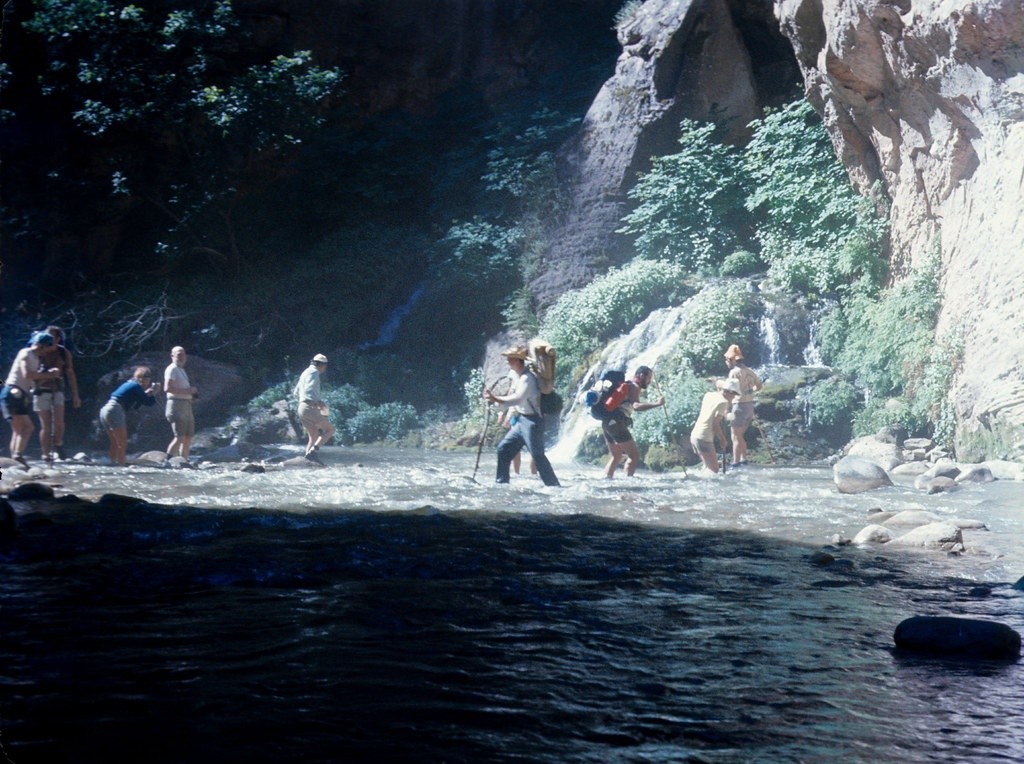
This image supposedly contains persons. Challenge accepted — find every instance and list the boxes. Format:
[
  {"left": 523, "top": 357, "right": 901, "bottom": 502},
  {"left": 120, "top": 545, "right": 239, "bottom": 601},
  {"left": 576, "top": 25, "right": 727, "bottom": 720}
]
[
  {"left": 601, "top": 366, "right": 665, "bottom": 478},
  {"left": 163, "top": 346, "right": 198, "bottom": 460},
  {"left": 690, "top": 378, "right": 740, "bottom": 473},
  {"left": 99, "top": 367, "right": 162, "bottom": 465},
  {"left": 706, "top": 344, "right": 763, "bottom": 467},
  {"left": 294, "top": 354, "right": 335, "bottom": 467},
  {"left": 0, "top": 326, "right": 81, "bottom": 467},
  {"left": 486, "top": 346, "right": 561, "bottom": 486}
]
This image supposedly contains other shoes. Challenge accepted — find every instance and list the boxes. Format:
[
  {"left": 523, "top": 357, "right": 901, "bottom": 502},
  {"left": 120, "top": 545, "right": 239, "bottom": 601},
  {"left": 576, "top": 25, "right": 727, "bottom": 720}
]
[
  {"left": 305, "top": 452, "right": 325, "bottom": 467},
  {"left": 12, "top": 455, "right": 30, "bottom": 470}
]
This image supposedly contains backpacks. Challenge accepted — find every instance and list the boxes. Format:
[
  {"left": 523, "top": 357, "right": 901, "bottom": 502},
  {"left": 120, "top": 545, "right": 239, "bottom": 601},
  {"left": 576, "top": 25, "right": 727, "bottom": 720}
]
[
  {"left": 531, "top": 338, "right": 562, "bottom": 415},
  {"left": 581, "top": 368, "right": 624, "bottom": 419}
]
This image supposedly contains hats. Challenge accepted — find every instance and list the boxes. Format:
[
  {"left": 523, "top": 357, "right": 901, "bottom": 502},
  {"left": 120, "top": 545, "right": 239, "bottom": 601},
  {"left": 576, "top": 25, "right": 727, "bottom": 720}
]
[
  {"left": 501, "top": 345, "right": 528, "bottom": 361},
  {"left": 45, "top": 326, "right": 63, "bottom": 337},
  {"left": 718, "top": 380, "right": 740, "bottom": 396},
  {"left": 312, "top": 353, "right": 330, "bottom": 366},
  {"left": 723, "top": 345, "right": 745, "bottom": 360},
  {"left": 28, "top": 331, "right": 54, "bottom": 346}
]
